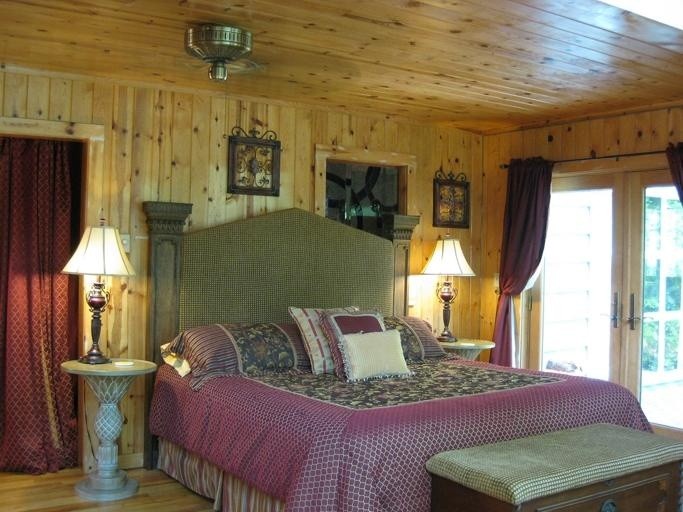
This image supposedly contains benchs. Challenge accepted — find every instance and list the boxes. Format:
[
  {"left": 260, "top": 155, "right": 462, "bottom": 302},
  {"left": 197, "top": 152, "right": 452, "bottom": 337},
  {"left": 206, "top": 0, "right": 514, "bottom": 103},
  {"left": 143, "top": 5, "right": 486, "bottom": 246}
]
[{"left": 422, "top": 421, "right": 681, "bottom": 511}]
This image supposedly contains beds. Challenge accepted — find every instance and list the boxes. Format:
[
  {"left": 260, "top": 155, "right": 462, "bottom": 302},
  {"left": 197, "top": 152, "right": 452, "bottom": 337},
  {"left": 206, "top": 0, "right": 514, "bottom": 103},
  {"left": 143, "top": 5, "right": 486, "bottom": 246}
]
[{"left": 143, "top": 201, "right": 649, "bottom": 510}]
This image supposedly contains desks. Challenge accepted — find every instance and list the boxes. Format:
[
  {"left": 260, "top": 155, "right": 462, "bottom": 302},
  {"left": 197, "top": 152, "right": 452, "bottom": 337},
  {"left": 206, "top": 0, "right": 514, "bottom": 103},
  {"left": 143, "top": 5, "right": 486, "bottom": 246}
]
[
  {"left": 437, "top": 337, "right": 496, "bottom": 363},
  {"left": 59, "top": 355, "right": 160, "bottom": 500}
]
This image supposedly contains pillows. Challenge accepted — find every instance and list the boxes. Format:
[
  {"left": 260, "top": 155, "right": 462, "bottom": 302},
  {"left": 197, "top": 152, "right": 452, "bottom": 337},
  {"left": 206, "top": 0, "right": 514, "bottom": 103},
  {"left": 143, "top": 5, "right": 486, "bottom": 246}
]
[
  {"left": 286, "top": 302, "right": 361, "bottom": 376},
  {"left": 161, "top": 320, "right": 312, "bottom": 392},
  {"left": 381, "top": 314, "right": 460, "bottom": 364},
  {"left": 318, "top": 305, "right": 389, "bottom": 384},
  {"left": 337, "top": 327, "right": 414, "bottom": 383}
]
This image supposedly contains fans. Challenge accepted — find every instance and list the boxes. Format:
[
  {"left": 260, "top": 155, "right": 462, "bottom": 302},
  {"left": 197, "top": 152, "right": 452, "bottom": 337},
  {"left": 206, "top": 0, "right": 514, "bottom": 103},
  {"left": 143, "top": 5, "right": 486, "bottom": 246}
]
[{"left": 150, "top": 20, "right": 359, "bottom": 105}]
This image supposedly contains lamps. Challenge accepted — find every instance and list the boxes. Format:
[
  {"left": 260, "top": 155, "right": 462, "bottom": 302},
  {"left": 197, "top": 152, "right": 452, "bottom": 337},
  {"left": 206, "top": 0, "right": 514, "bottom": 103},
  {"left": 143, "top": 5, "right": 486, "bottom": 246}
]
[
  {"left": 61, "top": 208, "right": 140, "bottom": 365},
  {"left": 419, "top": 230, "right": 477, "bottom": 342}
]
